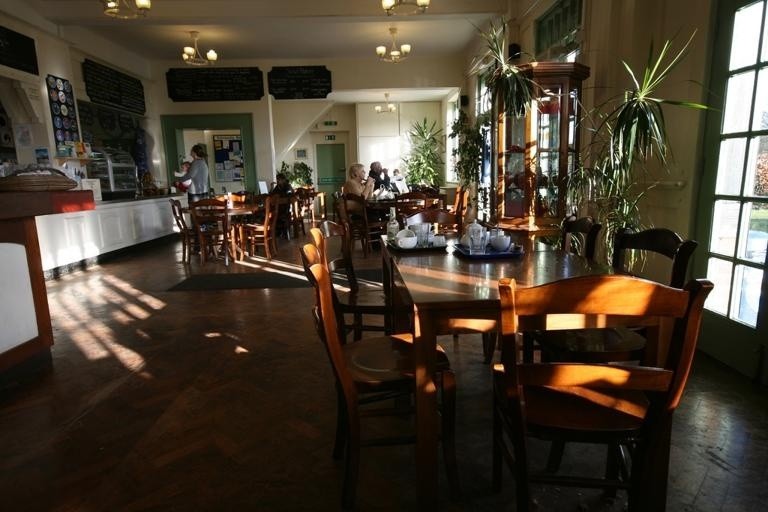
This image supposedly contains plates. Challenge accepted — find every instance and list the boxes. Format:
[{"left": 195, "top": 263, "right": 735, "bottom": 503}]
[{"left": 48, "top": 76, "right": 80, "bottom": 142}]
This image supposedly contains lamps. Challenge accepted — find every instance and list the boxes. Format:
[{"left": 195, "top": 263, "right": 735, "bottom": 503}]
[
  {"left": 382, "top": 0, "right": 430, "bottom": 16},
  {"left": 374, "top": 93, "right": 396, "bottom": 114},
  {"left": 103, "top": 0, "right": 152, "bottom": 20},
  {"left": 376, "top": 28, "right": 412, "bottom": 65},
  {"left": 182, "top": 31, "right": 218, "bottom": 67}
]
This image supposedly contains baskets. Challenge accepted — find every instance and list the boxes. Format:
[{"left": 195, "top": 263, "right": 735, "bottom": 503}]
[{"left": 0, "top": 167, "right": 77, "bottom": 191}]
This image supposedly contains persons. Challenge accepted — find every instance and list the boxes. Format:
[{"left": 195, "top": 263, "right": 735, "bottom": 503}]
[
  {"left": 172, "top": 144, "right": 208, "bottom": 255},
  {"left": 270, "top": 173, "right": 294, "bottom": 195},
  {"left": 173, "top": 162, "right": 192, "bottom": 192},
  {"left": 343, "top": 164, "right": 383, "bottom": 252},
  {"left": 394, "top": 169, "right": 405, "bottom": 183},
  {"left": 369, "top": 162, "right": 390, "bottom": 191}
]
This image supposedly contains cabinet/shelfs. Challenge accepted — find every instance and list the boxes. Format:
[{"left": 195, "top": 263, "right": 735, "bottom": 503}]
[
  {"left": 90, "top": 145, "right": 139, "bottom": 200},
  {"left": 484, "top": 62, "right": 590, "bottom": 237}
]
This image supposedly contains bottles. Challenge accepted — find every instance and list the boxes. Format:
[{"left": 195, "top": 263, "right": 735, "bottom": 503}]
[
  {"left": 385, "top": 206, "right": 400, "bottom": 245},
  {"left": 151, "top": 175, "right": 161, "bottom": 189},
  {"left": 226, "top": 191, "right": 234, "bottom": 210},
  {"left": 71, "top": 148, "right": 77, "bottom": 159}
]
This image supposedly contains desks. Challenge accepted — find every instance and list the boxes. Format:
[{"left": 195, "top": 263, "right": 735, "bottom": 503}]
[
  {"left": 378, "top": 233, "right": 673, "bottom": 509},
  {"left": 1, "top": 190, "right": 96, "bottom": 376}
]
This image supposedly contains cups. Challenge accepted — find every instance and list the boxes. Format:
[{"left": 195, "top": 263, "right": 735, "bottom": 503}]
[
  {"left": 161, "top": 188, "right": 169, "bottom": 195},
  {"left": 469, "top": 228, "right": 487, "bottom": 255},
  {"left": 460, "top": 219, "right": 523, "bottom": 253},
  {"left": 393, "top": 222, "right": 446, "bottom": 250}
]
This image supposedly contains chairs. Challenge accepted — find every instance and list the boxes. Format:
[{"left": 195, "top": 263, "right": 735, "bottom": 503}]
[
  {"left": 331, "top": 185, "right": 472, "bottom": 257},
  {"left": 520, "top": 226, "right": 700, "bottom": 463},
  {"left": 490, "top": 273, "right": 714, "bottom": 511},
  {"left": 307, "top": 263, "right": 463, "bottom": 509},
  {"left": 481, "top": 212, "right": 603, "bottom": 361},
  {"left": 298, "top": 217, "right": 429, "bottom": 343},
  {"left": 171, "top": 185, "right": 329, "bottom": 265}
]
